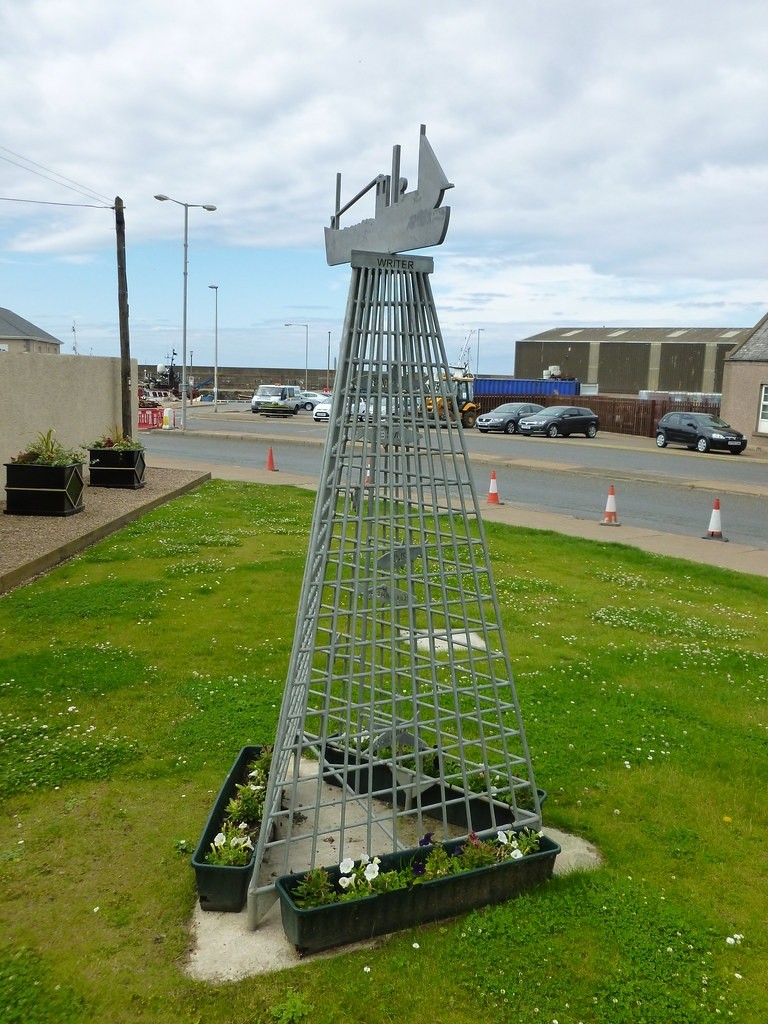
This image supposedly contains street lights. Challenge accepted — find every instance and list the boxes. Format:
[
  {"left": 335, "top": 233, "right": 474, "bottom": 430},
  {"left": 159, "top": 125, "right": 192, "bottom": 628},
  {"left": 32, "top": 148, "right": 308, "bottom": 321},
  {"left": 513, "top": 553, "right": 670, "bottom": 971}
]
[
  {"left": 327, "top": 331, "right": 332, "bottom": 387},
  {"left": 284, "top": 323, "right": 309, "bottom": 392},
  {"left": 153, "top": 193, "right": 217, "bottom": 431},
  {"left": 476, "top": 328, "right": 485, "bottom": 378},
  {"left": 207, "top": 285, "right": 219, "bottom": 413}
]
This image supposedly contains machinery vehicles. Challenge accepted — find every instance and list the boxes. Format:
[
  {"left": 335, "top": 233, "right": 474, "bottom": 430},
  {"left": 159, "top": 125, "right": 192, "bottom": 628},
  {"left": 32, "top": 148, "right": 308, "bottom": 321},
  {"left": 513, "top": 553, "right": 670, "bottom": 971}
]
[
  {"left": 406, "top": 370, "right": 481, "bottom": 430},
  {"left": 130, "top": 348, "right": 215, "bottom": 409}
]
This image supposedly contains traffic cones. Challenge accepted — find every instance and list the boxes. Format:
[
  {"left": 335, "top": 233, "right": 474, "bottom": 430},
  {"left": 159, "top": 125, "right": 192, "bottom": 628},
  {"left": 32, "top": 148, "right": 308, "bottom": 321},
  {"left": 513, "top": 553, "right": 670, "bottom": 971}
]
[
  {"left": 265, "top": 446, "right": 280, "bottom": 471},
  {"left": 700, "top": 498, "right": 730, "bottom": 542},
  {"left": 598, "top": 484, "right": 622, "bottom": 526},
  {"left": 485, "top": 469, "right": 506, "bottom": 506}
]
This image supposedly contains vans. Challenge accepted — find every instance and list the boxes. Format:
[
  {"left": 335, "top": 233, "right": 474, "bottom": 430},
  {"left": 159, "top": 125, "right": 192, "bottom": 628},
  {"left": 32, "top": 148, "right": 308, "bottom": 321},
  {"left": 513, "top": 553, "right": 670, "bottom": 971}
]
[{"left": 250, "top": 383, "right": 302, "bottom": 415}]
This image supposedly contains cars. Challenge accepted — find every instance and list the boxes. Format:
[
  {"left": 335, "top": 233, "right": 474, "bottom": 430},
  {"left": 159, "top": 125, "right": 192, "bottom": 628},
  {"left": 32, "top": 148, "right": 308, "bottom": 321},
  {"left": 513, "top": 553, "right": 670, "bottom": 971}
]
[
  {"left": 517, "top": 405, "right": 600, "bottom": 438},
  {"left": 475, "top": 402, "right": 546, "bottom": 435},
  {"left": 362, "top": 393, "right": 421, "bottom": 423},
  {"left": 300, "top": 391, "right": 329, "bottom": 411},
  {"left": 313, "top": 395, "right": 367, "bottom": 422},
  {"left": 655, "top": 411, "right": 748, "bottom": 455}
]
